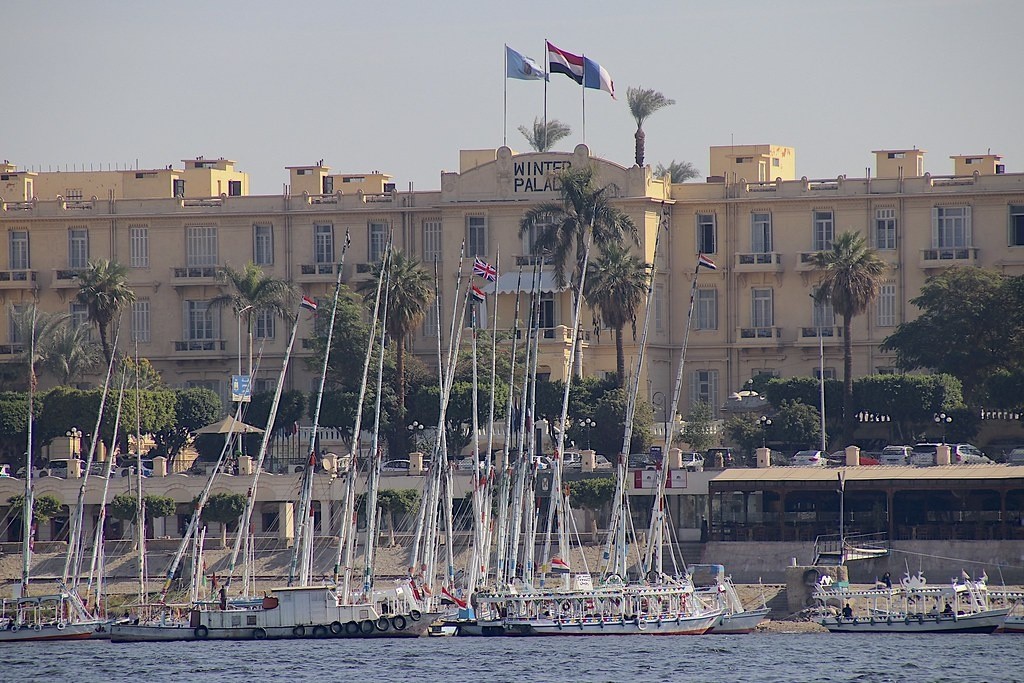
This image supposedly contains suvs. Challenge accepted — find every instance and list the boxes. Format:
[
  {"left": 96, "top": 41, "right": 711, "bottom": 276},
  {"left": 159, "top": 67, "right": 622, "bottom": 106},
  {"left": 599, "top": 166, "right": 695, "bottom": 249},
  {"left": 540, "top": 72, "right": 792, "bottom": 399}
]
[
  {"left": 877, "top": 443, "right": 995, "bottom": 466},
  {"left": 114, "top": 459, "right": 154, "bottom": 477},
  {"left": 50, "top": 458, "right": 87, "bottom": 478},
  {"left": 458, "top": 458, "right": 473, "bottom": 469}
]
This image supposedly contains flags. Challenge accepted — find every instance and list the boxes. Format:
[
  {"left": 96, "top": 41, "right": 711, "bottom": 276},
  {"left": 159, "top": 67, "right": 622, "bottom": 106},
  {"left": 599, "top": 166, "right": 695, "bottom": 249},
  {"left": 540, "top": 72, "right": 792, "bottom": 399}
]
[
  {"left": 546, "top": 41, "right": 584, "bottom": 85},
  {"left": 962, "top": 571, "right": 970, "bottom": 579},
  {"left": 551, "top": 559, "right": 570, "bottom": 573},
  {"left": 506, "top": 46, "right": 550, "bottom": 83},
  {"left": 473, "top": 258, "right": 496, "bottom": 282},
  {"left": 699, "top": 254, "right": 717, "bottom": 270},
  {"left": 468, "top": 284, "right": 485, "bottom": 303},
  {"left": 584, "top": 57, "right": 617, "bottom": 101},
  {"left": 511, "top": 397, "right": 530, "bottom": 432},
  {"left": 301, "top": 296, "right": 317, "bottom": 311},
  {"left": 442, "top": 588, "right": 467, "bottom": 610}
]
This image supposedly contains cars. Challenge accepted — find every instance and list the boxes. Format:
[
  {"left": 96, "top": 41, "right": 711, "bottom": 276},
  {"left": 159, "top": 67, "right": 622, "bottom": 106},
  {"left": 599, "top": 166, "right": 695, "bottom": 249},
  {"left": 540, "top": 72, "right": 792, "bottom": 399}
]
[
  {"left": 791, "top": 450, "right": 830, "bottom": 467},
  {"left": 627, "top": 454, "right": 650, "bottom": 468},
  {"left": 1006, "top": 447, "right": 1024, "bottom": 466},
  {"left": 378, "top": 460, "right": 429, "bottom": 473},
  {"left": 645, "top": 461, "right": 663, "bottom": 471},
  {"left": 567, "top": 454, "right": 612, "bottom": 469},
  {"left": 472, "top": 461, "right": 494, "bottom": 470},
  {"left": 681, "top": 452, "right": 703, "bottom": 472},
  {"left": 510, "top": 456, "right": 549, "bottom": 469},
  {"left": 828, "top": 451, "right": 876, "bottom": 465},
  {"left": 748, "top": 450, "right": 789, "bottom": 468}
]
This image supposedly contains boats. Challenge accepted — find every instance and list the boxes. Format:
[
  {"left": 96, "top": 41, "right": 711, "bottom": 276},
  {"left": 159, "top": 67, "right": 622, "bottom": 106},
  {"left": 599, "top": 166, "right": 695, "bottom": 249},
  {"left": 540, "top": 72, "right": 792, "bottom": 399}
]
[{"left": 781, "top": 471, "right": 1024, "bottom": 635}]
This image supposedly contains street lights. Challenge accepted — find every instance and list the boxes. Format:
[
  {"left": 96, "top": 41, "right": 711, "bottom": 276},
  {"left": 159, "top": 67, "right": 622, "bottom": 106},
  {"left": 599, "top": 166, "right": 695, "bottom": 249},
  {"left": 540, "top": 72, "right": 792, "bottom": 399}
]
[
  {"left": 756, "top": 416, "right": 772, "bottom": 448},
  {"left": 408, "top": 421, "right": 423, "bottom": 452},
  {"left": 653, "top": 391, "right": 667, "bottom": 446},
  {"left": 580, "top": 418, "right": 596, "bottom": 450},
  {"left": 935, "top": 413, "right": 952, "bottom": 445},
  {"left": 66, "top": 427, "right": 82, "bottom": 459}
]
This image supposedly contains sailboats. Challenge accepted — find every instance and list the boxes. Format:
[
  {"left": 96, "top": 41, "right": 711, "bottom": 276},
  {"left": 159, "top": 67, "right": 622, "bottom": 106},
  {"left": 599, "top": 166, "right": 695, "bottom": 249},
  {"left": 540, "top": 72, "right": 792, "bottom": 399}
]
[{"left": 0, "top": 183, "right": 788, "bottom": 642}]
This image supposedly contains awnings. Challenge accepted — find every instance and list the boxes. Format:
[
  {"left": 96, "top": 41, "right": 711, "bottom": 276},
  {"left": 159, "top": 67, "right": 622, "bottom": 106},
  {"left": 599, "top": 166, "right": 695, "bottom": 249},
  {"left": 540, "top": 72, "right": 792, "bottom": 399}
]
[
  {"left": 175, "top": 503, "right": 192, "bottom": 514},
  {"left": 260, "top": 503, "right": 279, "bottom": 513},
  {"left": 479, "top": 272, "right": 574, "bottom": 295}
]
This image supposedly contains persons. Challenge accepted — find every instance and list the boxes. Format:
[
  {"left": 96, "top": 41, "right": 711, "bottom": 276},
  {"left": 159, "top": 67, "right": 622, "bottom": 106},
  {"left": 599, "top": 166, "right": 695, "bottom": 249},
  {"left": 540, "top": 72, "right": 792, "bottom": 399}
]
[
  {"left": 882, "top": 571, "right": 891, "bottom": 588},
  {"left": 943, "top": 603, "right": 952, "bottom": 613},
  {"left": 930, "top": 605, "right": 940, "bottom": 618},
  {"left": 180, "top": 519, "right": 189, "bottom": 537},
  {"left": 843, "top": 603, "right": 853, "bottom": 620},
  {"left": 219, "top": 585, "right": 227, "bottom": 611}
]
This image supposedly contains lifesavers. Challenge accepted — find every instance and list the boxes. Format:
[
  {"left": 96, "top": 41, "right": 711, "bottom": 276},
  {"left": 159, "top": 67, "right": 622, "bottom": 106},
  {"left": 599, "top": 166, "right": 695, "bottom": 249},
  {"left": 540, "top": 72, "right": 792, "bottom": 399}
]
[
  {"left": 359, "top": 619, "right": 375, "bottom": 634},
  {"left": 410, "top": 609, "right": 421, "bottom": 621},
  {"left": 11, "top": 626, "right": 17, "bottom": 633},
  {"left": 293, "top": 625, "right": 306, "bottom": 637},
  {"left": 34, "top": 624, "right": 41, "bottom": 632},
  {"left": 562, "top": 603, "right": 570, "bottom": 611},
  {"left": 57, "top": 623, "right": 66, "bottom": 631},
  {"left": 194, "top": 625, "right": 209, "bottom": 639},
  {"left": 646, "top": 570, "right": 660, "bottom": 584},
  {"left": 313, "top": 625, "right": 329, "bottom": 638},
  {"left": 638, "top": 619, "right": 648, "bottom": 631},
  {"left": 345, "top": 621, "right": 359, "bottom": 636},
  {"left": 253, "top": 626, "right": 267, "bottom": 640},
  {"left": 375, "top": 616, "right": 390, "bottom": 631},
  {"left": 803, "top": 568, "right": 821, "bottom": 587},
  {"left": 392, "top": 615, "right": 406, "bottom": 631},
  {"left": 330, "top": 621, "right": 343, "bottom": 634}
]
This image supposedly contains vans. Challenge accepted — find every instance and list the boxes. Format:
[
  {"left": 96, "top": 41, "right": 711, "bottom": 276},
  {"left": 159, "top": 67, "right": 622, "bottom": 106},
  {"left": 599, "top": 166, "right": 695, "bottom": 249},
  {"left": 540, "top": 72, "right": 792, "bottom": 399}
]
[
  {"left": 550, "top": 452, "right": 581, "bottom": 469},
  {"left": 338, "top": 448, "right": 373, "bottom": 472},
  {"left": 0, "top": 464, "right": 13, "bottom": 477},
  {"left": 703, "top": 447, "right": 738, "bottom": 469}
]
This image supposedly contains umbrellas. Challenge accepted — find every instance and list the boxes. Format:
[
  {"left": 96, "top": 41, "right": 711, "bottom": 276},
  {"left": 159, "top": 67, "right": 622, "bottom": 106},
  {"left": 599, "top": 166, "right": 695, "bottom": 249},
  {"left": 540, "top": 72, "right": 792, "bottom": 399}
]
[{"left": 190, "top": 415, "right": 266, "bottom": 467}]
